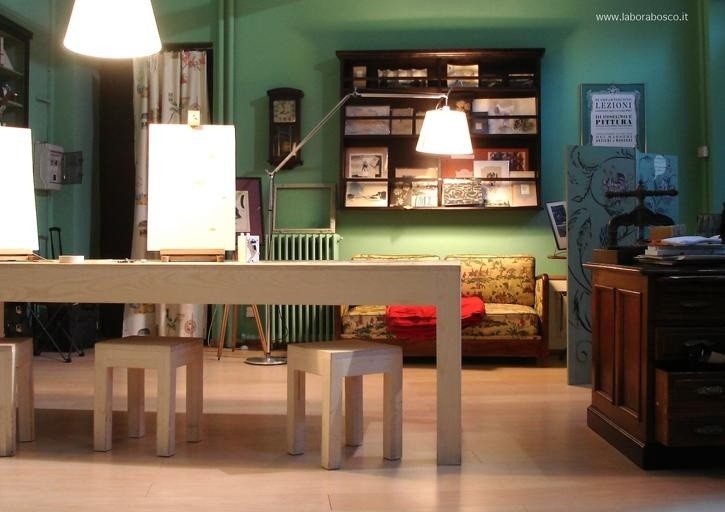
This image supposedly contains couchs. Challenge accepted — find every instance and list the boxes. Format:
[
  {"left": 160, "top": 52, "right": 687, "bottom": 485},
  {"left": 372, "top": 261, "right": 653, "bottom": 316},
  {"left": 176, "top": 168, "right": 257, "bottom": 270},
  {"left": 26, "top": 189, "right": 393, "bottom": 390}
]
[{"left": 339, "top": 254, "right": 549, "bottom": 366}]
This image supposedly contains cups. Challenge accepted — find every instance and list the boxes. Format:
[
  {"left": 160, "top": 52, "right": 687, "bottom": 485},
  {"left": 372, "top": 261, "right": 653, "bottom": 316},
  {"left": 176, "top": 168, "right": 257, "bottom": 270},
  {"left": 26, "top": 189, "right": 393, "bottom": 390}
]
[{"left": 238, "top": 235, "right": 260, "bottom": 265}]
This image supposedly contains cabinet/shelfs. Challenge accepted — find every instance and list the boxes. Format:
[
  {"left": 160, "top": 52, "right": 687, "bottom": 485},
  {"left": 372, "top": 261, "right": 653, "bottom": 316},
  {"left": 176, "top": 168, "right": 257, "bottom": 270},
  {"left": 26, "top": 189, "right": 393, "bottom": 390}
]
[
  {"left": 0, "top": 15, "right": 34, "bottom": 128},
  {"left": 339, "top": 48, "right": 547, "bottom": 212},
  {"left": 583, "top": 263, "right": 724, "bottom": 469}
]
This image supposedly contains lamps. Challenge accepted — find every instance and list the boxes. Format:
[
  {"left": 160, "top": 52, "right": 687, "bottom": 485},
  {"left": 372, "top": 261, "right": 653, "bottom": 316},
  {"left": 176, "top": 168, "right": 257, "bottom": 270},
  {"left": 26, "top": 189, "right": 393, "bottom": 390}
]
[
  {"left": 62, "top": 0, "right": 163, "bottom": 61},
  {"left": 0, "top": 127, "right": 40, "bottom": 255},
  {"left": 245, "top": 88, "right": 474, "bottom": 366}
]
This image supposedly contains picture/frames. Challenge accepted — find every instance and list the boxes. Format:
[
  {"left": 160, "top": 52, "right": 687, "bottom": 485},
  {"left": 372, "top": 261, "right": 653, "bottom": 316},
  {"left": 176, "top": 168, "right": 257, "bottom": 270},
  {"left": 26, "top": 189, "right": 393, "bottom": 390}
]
[
  {"left": 545, "top": 201, "right": 568, "bottom": 250},
  {"left": 236, "top": 178, "right": 264, "bottom": 246}
]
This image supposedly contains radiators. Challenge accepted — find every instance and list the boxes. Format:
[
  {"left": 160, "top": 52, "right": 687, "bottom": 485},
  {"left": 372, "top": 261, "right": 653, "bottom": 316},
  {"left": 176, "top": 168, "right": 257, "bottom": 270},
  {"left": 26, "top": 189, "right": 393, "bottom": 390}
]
[{"left": 263, "top": 235, "right": 343, "bottom": 345}]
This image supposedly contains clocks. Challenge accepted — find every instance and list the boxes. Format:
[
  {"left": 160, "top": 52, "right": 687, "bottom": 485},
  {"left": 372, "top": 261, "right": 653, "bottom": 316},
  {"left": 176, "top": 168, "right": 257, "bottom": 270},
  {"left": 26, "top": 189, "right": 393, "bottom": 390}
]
[{"left": 267, "top": 87, "right": 305, "bottom": 169}]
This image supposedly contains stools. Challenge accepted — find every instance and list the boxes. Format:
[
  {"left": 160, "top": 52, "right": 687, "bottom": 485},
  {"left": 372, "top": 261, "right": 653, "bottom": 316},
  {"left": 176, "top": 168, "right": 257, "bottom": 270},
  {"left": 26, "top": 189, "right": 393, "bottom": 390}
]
[
  {"left": 286, "top": 338, "right": 403, "bottom": 471},
  {"left": 94, "top": 336, "right": 204, "bottom": 457},
  {"left": 0, "top": 337, "right": 35, "bottom": 457}
]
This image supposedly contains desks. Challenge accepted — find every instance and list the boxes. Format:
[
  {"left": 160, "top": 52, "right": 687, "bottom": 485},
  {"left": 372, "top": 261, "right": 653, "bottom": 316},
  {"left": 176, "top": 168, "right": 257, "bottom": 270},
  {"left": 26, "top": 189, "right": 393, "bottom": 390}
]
[{"left": 0, "top": 258, "right": 462, "bottom": 469}]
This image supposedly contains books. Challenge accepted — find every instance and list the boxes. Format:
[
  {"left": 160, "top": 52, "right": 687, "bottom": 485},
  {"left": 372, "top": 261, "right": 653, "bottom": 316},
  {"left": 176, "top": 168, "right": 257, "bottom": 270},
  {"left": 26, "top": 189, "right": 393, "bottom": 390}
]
[{"left": 634, "top": 240, "right": 725, "bottom": 270}]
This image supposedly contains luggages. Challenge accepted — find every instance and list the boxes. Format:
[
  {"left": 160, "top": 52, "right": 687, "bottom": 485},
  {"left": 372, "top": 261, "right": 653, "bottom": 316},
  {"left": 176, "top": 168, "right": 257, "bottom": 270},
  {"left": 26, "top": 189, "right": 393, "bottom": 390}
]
[{"left": 46, "top": 227, "right": 98, "bottom": 352}]
